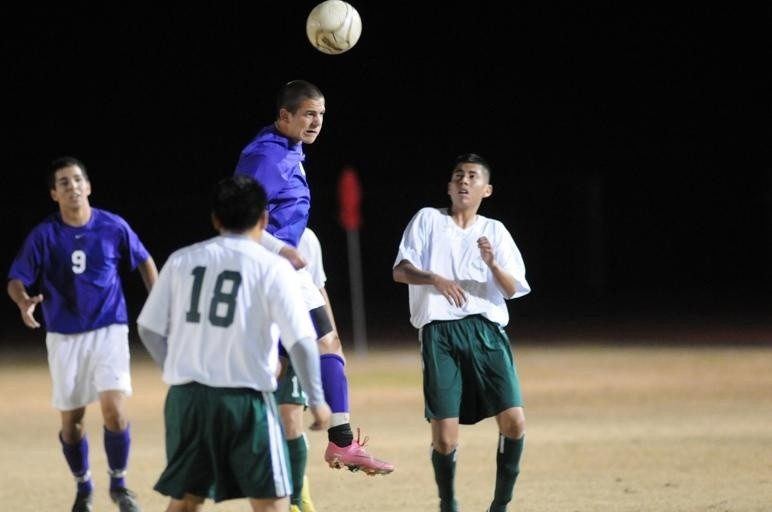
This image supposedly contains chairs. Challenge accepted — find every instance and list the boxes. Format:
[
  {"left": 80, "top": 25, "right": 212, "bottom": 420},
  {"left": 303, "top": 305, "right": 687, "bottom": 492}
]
[
  {"left": 109, "top": 483, "right": 139, "bottom": 512},
  {"left": 325, "top": 428, "right": 395, "bottom": 477},
  {"left": 71, "top": 489, "right": 93, "bottom": 511}
]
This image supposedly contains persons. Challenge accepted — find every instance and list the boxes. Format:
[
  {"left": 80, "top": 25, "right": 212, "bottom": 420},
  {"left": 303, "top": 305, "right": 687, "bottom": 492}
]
[
  {"left": 393, "top": 152, "right": 533, "bottom": 511},
  {"left": 5, "top": 153, "right": 159, "bottom": 512},
  {"left": 135, "top": 174, "right": 333, "bottom": 512},
  {"left": 278, "top": 227, "right": 330, "bottom": 512},
  {"left": 232, "top": 79, "right": 393, "bottom": 477}
]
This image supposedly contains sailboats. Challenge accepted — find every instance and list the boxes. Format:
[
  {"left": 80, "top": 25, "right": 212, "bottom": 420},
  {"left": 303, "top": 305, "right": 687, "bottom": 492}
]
[{"left": 307, "top": 0, "right": 363, "bottom": 54}]
